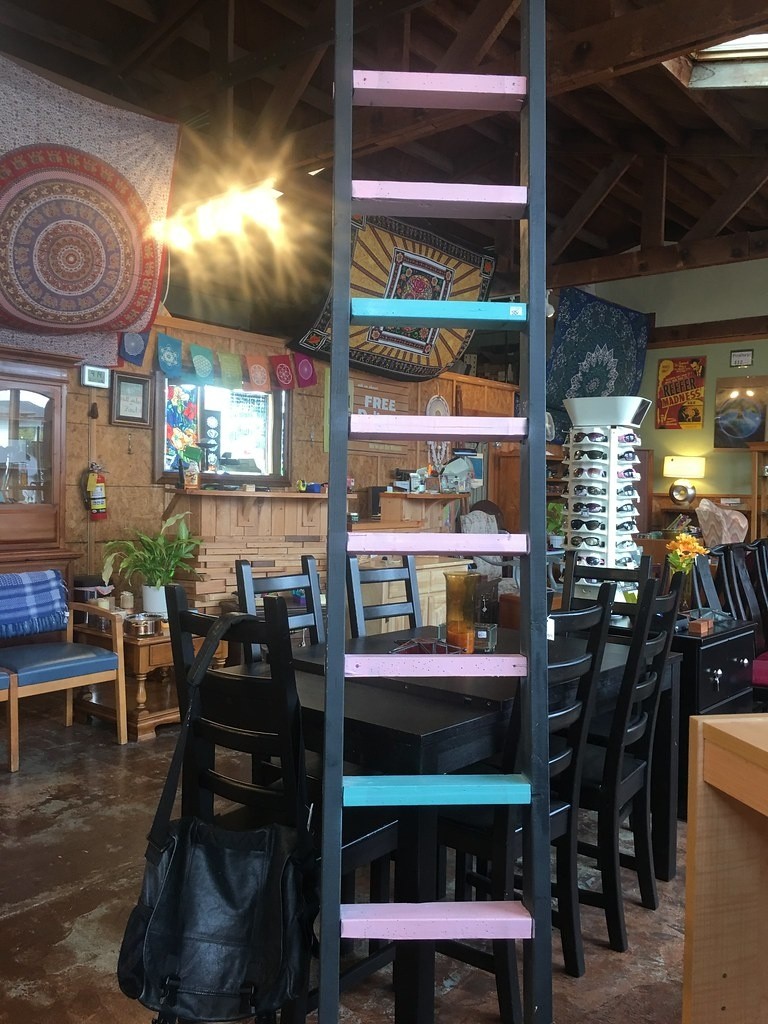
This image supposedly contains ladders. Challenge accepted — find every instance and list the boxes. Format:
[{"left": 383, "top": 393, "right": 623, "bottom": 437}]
[{"left": 318, "top": 0, "right": 553, "bottom": 1024}]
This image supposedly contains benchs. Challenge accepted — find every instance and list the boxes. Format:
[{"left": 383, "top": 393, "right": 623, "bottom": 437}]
[{"left": 0, "top": 568, "right": 127, "bottom": 773}]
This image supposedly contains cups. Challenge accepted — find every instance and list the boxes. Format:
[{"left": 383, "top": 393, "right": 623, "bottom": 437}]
[{"left": 443, "top": 571, "right": 481, "bottom": 653}]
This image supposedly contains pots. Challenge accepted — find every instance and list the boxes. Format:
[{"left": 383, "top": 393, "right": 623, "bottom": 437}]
[{"left": 124, "top": 613, "right": 163, "bottom": 637}]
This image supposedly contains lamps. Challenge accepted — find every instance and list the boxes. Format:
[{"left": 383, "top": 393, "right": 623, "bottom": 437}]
[{"left": 663, "top": 456, "right": 705, "bottom": 508}]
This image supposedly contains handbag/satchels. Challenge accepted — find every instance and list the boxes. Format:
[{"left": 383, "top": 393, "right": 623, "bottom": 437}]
[{"left": 116, "top": 817, "right": 322, "bottom": 1022}]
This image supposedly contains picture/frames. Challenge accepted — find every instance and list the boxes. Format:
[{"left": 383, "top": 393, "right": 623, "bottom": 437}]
[
  {"left": 108, "top": 369, "right": 156, "bottom": 429},
  {"left": 152, "top": 370, "right": 294, "bottom": 488}
]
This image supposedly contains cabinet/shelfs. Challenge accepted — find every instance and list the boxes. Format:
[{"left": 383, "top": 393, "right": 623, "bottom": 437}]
[
  {"left": 660, "top": 440, "right": 768, "bottom": 548},
  {"left": 656, "top": 618, "right": 757, "bottom": 819},
  {"left": 344, "top": 558, "right": 474, "bottom": 640},
  {"left": 496, "top": 451, "right": 568, "bottom": 532}
]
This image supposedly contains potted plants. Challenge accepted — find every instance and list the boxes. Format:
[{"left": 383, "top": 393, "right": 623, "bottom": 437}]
[{"left": 100, "top": 509, "right": 209, "bottom": 616}]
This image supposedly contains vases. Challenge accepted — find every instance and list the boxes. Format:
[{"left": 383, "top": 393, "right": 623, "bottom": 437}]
[{"left": 670, "top": 569, "right": 692, "bottom": 609}]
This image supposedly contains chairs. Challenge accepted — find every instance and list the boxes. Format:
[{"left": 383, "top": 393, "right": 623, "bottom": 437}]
[{"left": 162, "top": 537, "right": 768, "bottom": 1024}]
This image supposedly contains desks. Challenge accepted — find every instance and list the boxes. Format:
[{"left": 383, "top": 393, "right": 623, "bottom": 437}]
[
  {"left": 682, "top": 715, "right": 768, "bottom": 1024},
  {"left": 217, "top": 626, "right": 685, "bottom": 1024},
  {"left": 72, "top": 622, "right": 229, "bottom": 743}
]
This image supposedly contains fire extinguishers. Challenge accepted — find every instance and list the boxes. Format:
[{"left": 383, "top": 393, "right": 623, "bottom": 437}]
[{"left": 81, "top": 463, "right": 110, "bottom": 521}]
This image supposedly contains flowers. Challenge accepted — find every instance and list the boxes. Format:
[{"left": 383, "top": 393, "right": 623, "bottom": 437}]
[{"left": 666, "top": 533, "right": 709, "bottom": 577}]
